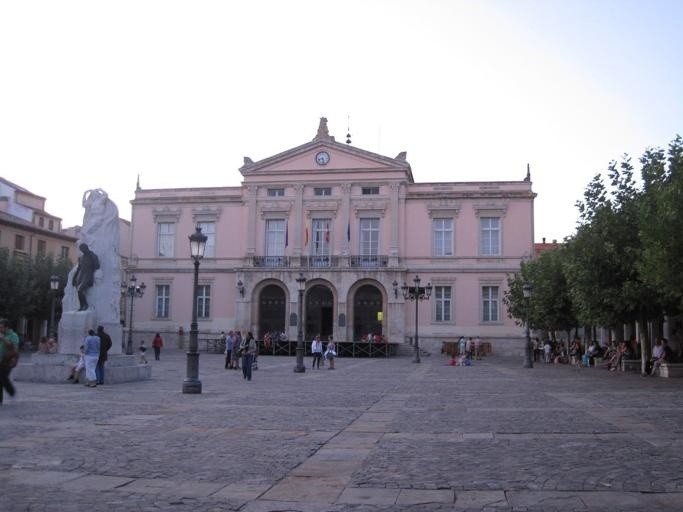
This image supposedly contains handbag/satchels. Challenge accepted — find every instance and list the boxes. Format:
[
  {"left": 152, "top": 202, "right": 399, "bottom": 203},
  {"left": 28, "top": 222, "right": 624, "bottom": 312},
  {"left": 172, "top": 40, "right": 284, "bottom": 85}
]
[
  {"left": 327, "top": 348, "right": 334, "bottom": 360},
  {"left": 319, "top": 358, "right": 324, "bottom": 366},
  {"left": 235, "top": 348, "right": 243, "bottom": 358},
  {"left": 0, "top": 342, "right": 19, "bottom": 368}
]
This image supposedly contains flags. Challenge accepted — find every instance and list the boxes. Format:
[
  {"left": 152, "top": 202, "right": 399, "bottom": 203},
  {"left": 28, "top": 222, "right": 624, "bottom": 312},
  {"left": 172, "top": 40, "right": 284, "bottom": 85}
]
[
  {"left": 304, "top": 226, "right": 309, "bottom": 248},
  {"left": 325, "top": 223, "right": 330, "bottom": 243}
]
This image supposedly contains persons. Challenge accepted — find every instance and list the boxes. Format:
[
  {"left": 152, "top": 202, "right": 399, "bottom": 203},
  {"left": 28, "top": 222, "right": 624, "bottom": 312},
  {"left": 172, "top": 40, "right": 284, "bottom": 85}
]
[
  {"left": 81, "top": 188, "right": 108, "bottom": 247},
  {"left": 71, "top": 243, "right": 100, "bottom": 312},
  {"left": 0, "top": 318, "right": 19, "bottom": 404},
  {"left": 47, "top": 338, "right": 58, "bottom": 354},
  {"left": 311, "top": 336, "right": 322, "bottom": 370},
  {"left": 264, "top": 329, "right": 287, "bottom": 352},
  {"left": 138, "top": 340, "right": 148, "bottom": 364},
  {"left": 327, "top": 335, "right": 336, "bottom": 370},
  {"left": 449, "top": 334, "right": 482, "bottom": 367},
  {"left": 32, "top": 336, "right": 48, "bottom": 360},
  {"left": 360, "top": 333, "right": 389, "bottom": 343},
  {"left": 529, "top": 335, "right": 673, "bottom": 376},
  {"left": 152, "top": 333, "right": 164, "bottom": 361},
  {"left": 220, "top": 330, "right": 259, "bottom": 381},
  {"left": 67, "top": 325, "right": 112, "bottom": 387}
]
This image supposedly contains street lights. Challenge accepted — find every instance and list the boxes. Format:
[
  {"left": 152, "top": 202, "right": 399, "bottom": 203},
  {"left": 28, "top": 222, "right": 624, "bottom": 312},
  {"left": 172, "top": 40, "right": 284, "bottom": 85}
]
[
  {"left": 120, "top": 274, "right": 147, "bottom": 356},
  {"left": 182, "top": 221, "right": 208, "bottom": 397},
  {"left": 293, "top": 271, "right": 307, "bottom": 374},
  {"left": 48, "top": 275, "right": 60, "bottom": 341},
  {"left": 522, "top": 280, "right": 534, "bottom": 369},
  {"left": 400, "top": 275, "right": 432, "bottom": 363}
]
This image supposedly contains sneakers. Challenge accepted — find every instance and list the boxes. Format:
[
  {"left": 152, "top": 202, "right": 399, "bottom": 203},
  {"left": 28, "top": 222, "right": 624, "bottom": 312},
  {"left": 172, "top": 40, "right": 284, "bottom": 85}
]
[
  {"left": 67, "top": 375, "right": 74, "bottom": 380},
  {"left": 73, "top": 379, "right": 79, "bottom": 384}
]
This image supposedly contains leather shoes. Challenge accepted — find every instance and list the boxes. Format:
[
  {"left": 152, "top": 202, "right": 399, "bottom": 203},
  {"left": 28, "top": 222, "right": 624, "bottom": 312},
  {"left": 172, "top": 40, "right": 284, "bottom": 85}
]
[{"left": 85, "top": 380, "right": 104, "bottom": 387}]
